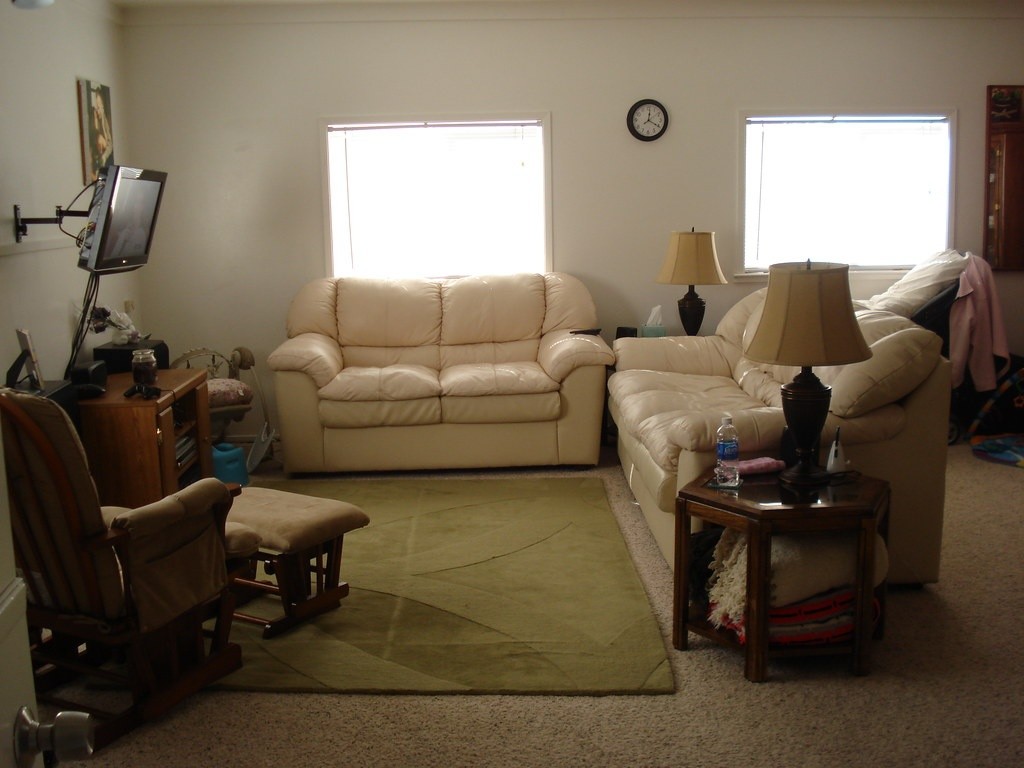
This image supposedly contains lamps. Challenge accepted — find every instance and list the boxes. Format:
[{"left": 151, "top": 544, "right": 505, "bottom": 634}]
[
  {"left": 656, "top": 226, "right": 728, "bottom": 336},
  {"left": 742, "top": 260, "right": 873, "bottom": 503}
]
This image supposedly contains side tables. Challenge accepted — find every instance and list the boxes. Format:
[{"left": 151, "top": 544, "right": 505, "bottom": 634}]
[{"left": 671, "top": 451, "right": 894, "bottom": 683}]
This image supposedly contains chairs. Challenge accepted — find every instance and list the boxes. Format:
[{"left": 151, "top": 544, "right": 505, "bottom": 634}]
[
  {"left": 870, "top": 248, "right": 970, "bottom": 355},
  {"left": 0, "top": 388, "right": 245, "bottom": 749}
]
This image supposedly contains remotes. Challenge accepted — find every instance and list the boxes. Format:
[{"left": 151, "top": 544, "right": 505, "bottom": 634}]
[{"left": 569, "top": 328, "right": 602, "bottom": 335}]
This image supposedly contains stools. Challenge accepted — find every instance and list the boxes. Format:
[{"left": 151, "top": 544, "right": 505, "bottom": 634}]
[{"left": 207, "top": 378, "right": 253, "bottom": 444}]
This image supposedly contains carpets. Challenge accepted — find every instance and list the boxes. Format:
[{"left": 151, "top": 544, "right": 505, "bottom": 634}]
[{"left": 198, "top": 474, "right": 676, "bottom": 696}]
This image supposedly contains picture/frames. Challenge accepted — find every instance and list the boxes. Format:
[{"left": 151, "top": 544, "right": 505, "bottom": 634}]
[{"left": 5, "top": 328, "right": 45, "bottom": 390}]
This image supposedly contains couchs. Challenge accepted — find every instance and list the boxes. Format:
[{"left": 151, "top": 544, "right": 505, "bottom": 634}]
[
  {"left": 265, "top": 272, "right": 616, "bottom": 476},
  {"left": 607, "top": 286, "right": 950, "bottom": 612}
]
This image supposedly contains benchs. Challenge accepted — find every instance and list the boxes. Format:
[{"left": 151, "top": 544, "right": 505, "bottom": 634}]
[{"left": 227, "top": 487, "right": 370, "bottom": 639}]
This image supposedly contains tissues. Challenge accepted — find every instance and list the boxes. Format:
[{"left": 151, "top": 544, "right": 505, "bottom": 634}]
[{"left": 639, "top": 305, "right": 667, "bottom": 337}]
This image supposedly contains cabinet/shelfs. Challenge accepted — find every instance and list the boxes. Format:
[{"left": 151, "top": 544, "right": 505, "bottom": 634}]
[{"left": 58, "top": 369, "right": 212, "bottom": 509}]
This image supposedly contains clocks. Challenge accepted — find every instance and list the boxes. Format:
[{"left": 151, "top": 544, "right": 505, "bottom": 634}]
[{"left": 626, "top": 99, "right": 668, "bottom": 141}]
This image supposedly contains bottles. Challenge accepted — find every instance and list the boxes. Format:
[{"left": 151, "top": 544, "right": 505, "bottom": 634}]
[
  {"left": 715, "top": 417, "right": 740, "bottom": 486},
  {"left": 132, "top": 349, "right": 158, "bottom": 386}
]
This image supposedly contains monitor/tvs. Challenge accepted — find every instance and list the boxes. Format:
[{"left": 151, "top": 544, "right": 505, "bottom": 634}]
[{"left": 77, "top": 165, "right": 168, "bottom": 275}]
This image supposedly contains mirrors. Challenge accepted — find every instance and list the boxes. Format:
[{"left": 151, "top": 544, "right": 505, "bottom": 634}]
[{"left": 982, "top": 84, "right": 1024, "bottom": 272}]
